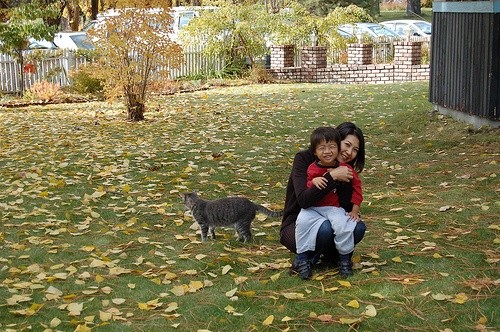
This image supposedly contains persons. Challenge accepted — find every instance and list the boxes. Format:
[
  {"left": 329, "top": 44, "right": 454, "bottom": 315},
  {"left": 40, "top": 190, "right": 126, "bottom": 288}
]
[
  {"left": 279, "top": 122, "right": 367, "bottom": 268},
  {"left": 295, "top": 127, "right": 363, "bottom": 281}
]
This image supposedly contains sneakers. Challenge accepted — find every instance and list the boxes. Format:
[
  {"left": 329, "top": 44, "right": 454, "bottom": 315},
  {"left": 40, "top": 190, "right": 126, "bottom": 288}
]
[
  {"left": 339, "top": 260, "right": 353, "bottom": 279},
  {"left": 298, "top": 261, "right": 312, "bottom": 279}
]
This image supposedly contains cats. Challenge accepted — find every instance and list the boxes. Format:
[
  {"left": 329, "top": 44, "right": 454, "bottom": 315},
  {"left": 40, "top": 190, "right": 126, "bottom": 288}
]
[{"left": 180, "top": 191, "right": 284, "bottom": 243}]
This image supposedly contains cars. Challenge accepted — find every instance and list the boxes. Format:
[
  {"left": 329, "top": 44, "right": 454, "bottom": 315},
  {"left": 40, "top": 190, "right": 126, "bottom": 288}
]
[{"left": 1, "top": 6, "right": 432, "bottom": 89}]
